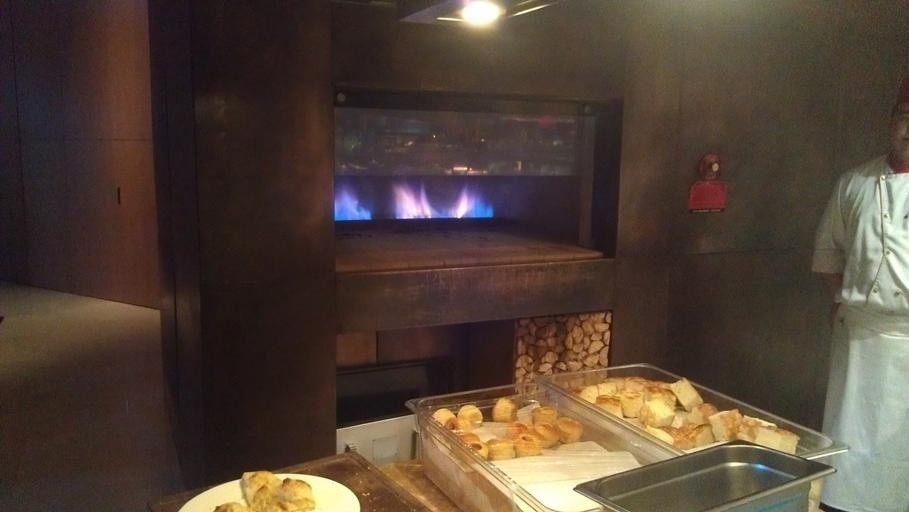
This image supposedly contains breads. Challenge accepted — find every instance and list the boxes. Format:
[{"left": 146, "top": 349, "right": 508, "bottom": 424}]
[
  {"left": 433, "top": 397, "right": 583, "bottom": 461},
  {"left": 214, "top": 470, "right": 315, "bottom": 512},
  {"left": 565, "top": 376, "right": 802, "bottom": 455}
]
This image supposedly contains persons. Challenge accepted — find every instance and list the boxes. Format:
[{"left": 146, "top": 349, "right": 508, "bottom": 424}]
[{"left": 810, "top": 74, "right": 909, "bottom": 512}]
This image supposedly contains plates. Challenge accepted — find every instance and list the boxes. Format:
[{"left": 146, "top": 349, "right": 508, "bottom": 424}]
[{"left": 177, "top": 473, "right": 362, "bottom": 512}]
